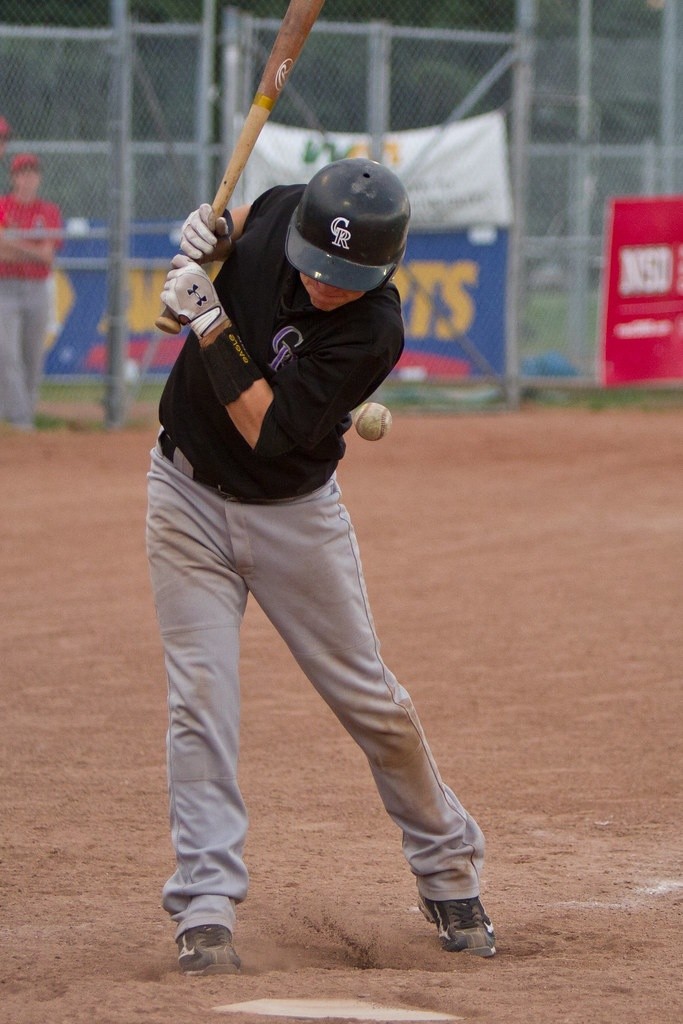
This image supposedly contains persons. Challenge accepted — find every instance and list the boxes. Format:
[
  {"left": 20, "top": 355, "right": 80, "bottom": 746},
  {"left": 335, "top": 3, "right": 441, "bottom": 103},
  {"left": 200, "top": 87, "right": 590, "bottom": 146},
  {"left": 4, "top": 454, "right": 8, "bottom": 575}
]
[
  {"left": 1, "top": 152, "right": 65, "bottom": 432},
  {"left": 143, "top": 164, "right": 500, "bottom": 971}
]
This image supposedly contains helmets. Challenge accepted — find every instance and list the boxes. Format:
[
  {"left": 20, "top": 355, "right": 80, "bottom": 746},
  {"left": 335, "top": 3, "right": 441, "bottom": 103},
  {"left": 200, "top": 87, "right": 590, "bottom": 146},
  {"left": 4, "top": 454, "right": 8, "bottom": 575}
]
[{"left": 284, "top": 156, "right": 412, "bottom": 294}]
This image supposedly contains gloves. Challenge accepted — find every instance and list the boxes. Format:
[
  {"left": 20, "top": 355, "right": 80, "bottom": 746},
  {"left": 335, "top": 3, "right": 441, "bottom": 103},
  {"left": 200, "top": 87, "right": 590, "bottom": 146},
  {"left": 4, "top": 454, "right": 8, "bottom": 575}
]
[
  {"left": 161, "top": 253, "right": 228, "bottom": 337},
  {"left": 178, "top": 204, "right": 234, "bottom": 264}
]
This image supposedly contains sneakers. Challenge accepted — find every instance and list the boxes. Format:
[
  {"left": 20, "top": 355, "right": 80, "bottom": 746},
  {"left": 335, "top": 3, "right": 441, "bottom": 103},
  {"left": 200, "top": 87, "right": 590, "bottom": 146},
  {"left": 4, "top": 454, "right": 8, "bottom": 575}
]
[
  {"left": 418, "top": 889, "right": 499, "bottom": 958},
  {"left": 176, "top": 924, "right": 241, "bottom": 976}
]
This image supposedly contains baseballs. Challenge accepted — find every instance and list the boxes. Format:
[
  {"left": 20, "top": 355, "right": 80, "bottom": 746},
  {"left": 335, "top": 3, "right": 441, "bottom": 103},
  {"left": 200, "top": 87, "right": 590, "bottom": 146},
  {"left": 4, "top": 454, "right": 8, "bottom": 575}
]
[{"left": 355, "top": 403, "right": 392, "bottom": 441}]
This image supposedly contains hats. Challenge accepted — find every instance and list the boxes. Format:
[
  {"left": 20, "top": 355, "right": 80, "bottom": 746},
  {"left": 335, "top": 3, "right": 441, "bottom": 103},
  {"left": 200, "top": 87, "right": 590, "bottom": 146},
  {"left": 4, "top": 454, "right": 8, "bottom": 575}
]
[{"left": 11, "top": 154, "right": 39, "bottom": 172}]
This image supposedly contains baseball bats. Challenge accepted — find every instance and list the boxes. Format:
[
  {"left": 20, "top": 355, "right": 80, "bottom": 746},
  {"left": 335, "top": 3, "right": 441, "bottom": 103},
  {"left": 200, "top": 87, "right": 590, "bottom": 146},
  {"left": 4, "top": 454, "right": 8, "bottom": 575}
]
[{"left": 155, "top": 0, "right": 324, "bottom": 334}]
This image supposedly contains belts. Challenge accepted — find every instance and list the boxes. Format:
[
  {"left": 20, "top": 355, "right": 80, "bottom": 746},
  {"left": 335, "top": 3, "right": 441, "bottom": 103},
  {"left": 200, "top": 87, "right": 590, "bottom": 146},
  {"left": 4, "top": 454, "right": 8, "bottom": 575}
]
[{"left": 157, "top": 431, "right": 238, "bottom": 497}]
